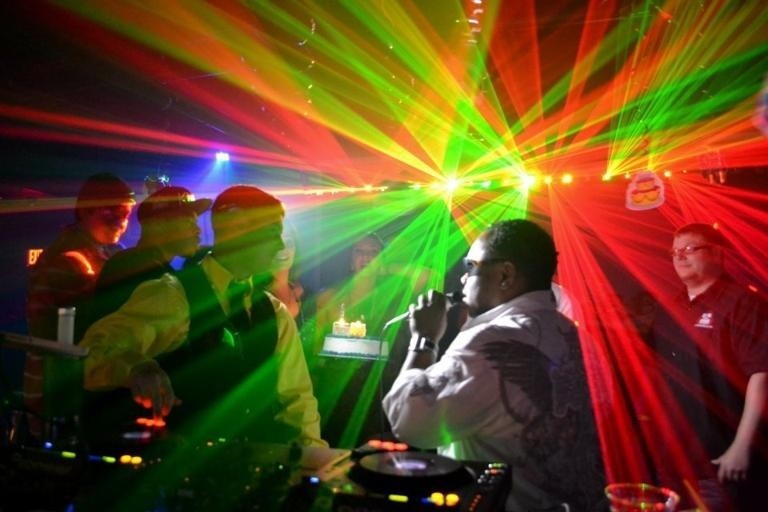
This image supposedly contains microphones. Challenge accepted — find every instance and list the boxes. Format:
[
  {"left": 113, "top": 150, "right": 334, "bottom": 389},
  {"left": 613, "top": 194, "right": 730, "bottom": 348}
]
[{"left": 386, "top": 289, "right": 464, "bottom": 326}]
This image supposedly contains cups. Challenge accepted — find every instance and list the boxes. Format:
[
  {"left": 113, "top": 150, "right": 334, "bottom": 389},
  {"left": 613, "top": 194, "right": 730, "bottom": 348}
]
[
  {"left": 601, "top": 480, "right": 684, "bottom": 512},
  {"left": 346, "top": 316, "right": 369, "bottom": 339},
  {"left": 333, "top": 319, "right": 350, "bottom": 337}
]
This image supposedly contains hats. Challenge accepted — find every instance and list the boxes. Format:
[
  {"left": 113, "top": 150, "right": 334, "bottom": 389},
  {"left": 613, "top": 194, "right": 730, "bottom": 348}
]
[{"left": 138, "top": 185, "right": 212, "bottom": 224}]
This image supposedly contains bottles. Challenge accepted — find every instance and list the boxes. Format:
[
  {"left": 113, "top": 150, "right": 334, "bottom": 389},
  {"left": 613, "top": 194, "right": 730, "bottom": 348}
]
[{"left": 36, "top": 306, "right": 87, "bottom": 447}]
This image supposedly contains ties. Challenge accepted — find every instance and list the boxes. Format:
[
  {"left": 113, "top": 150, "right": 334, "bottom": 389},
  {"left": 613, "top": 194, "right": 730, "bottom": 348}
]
[{"left": 230, "top": 282, "right": 253, "bottom": 367}]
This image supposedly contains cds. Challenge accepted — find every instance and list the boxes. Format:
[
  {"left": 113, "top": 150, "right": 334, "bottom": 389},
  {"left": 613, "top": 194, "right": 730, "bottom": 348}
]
[{"left": 360, "top": 450, "right": 463, "bottom": 478}]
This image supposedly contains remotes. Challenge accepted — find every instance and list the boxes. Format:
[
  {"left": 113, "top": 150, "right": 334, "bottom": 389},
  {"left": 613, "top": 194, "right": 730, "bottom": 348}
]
[{"left": 476, "top": 461, "right": 509, "bottom": 493}]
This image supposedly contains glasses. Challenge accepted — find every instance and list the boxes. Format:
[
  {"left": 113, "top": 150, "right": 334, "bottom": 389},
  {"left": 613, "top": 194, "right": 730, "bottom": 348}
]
[
  {"left": 462, "top": 257, "right": 505, "bottom": 274},
  {"left": 669, "top": 245, "right": 711, "bottom": 257}
]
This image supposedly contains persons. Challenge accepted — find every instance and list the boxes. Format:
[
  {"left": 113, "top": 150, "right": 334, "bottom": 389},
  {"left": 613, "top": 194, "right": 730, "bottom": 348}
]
[{"left": 23, "top": 172, "right": 767, "bottom": 512}]
